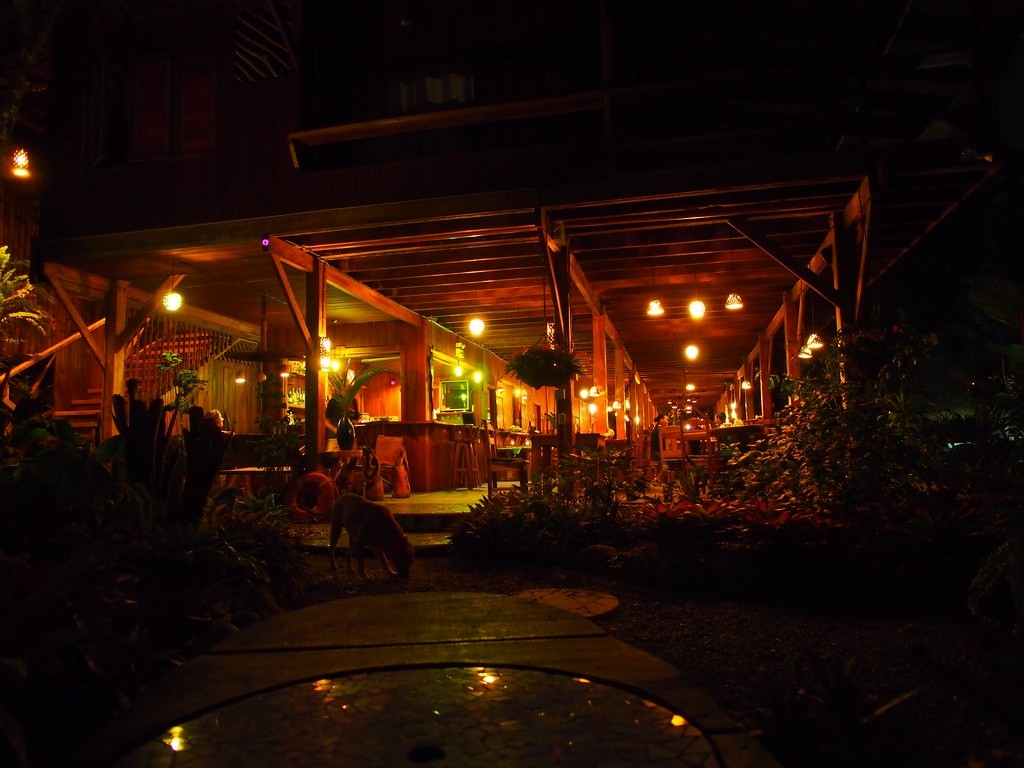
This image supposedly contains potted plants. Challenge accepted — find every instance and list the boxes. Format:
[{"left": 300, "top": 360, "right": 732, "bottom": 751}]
[
  {"left": 325, "top": 357, "right": 388, "bottom": 450},
  {"left": 505, "top": 345, "right": 588, "bottom": 390}
]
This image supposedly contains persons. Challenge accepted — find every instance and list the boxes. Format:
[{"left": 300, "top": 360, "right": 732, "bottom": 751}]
[
  {"left": 649, "top": 414, "right": 686, "bottom": 461},
  {"left": 714, "top": 413, "right": 735, "bottom": 454},
  {"left": 325, "top": 399, "right": 372, "bottom": 479}
]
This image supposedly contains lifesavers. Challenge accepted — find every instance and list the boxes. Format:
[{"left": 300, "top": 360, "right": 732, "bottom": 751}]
[{"left": 287, "top": 471, "right": 335, "bottom": 522}]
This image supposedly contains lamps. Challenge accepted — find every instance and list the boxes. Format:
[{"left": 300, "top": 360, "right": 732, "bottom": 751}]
[
  {"left": 279, "top": 364, "right": 289, "bottom": 378},
  {"left": 797, "top": 291, "right": 824, "bottom": 359},
  {"left": 688, "top": 273, "right": 705, "bottom": 318},
  {"left": 162, "top": 275, "right": 182, "bottom": 312},
  {"left": 646, "top": 268, "right": 664, "bottom": 316},
  {"left": 724, "top": 239, "right": 743, "bottom": 310},
  {"left": 236, "top": 370, "right": 246, "bottom": 383}
]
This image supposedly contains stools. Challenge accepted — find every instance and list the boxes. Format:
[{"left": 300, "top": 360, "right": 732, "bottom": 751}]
[{"left": 449, "top": 424, "right": 481, "bottom": 490}]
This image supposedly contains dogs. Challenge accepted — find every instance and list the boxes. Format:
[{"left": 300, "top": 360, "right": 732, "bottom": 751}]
[{"left": 329, "top": 491, "right": 415, "bottom": 580}]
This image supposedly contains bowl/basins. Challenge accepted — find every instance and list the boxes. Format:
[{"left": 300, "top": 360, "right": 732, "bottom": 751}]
[{"left": 723, "top": 423, "right": 732, "bottom": 428}]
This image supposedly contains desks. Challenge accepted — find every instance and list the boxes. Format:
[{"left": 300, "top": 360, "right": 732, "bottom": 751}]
[
  {"left": 529, "top": 434, "right": 607, "bottom": 496},
  {"left": 708, "top": 425, "right": 764, "bottom": 455},
  {"left": 606, "top": 440, "right": 626, "bottom": 451},
  {"left": 322, "top": 450, "right": 363, "bottom": 496},
  {"left": 216, "top": 467, "right": 292, "bottom": 497}
]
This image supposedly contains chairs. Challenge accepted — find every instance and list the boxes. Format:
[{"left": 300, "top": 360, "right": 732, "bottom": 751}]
[
  {"left": 354, "top": 434, "right": 410, "bottom": 501},
  {"left": 482, "top": 419, "right": 787, "bottom": 499}
]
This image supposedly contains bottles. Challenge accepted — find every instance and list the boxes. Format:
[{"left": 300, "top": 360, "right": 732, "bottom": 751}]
[
  {"left": 288, "top": 411, "right": 294, "bottom": 425},
  {"left": 288, "top": 387, "right": 305, "bottom": 407},
  {"left": 528, "top": 421, "right": 532, "bottom": 432},
  {"left": 290, "top": 362, "right": 306, "bottom": 376}
]
